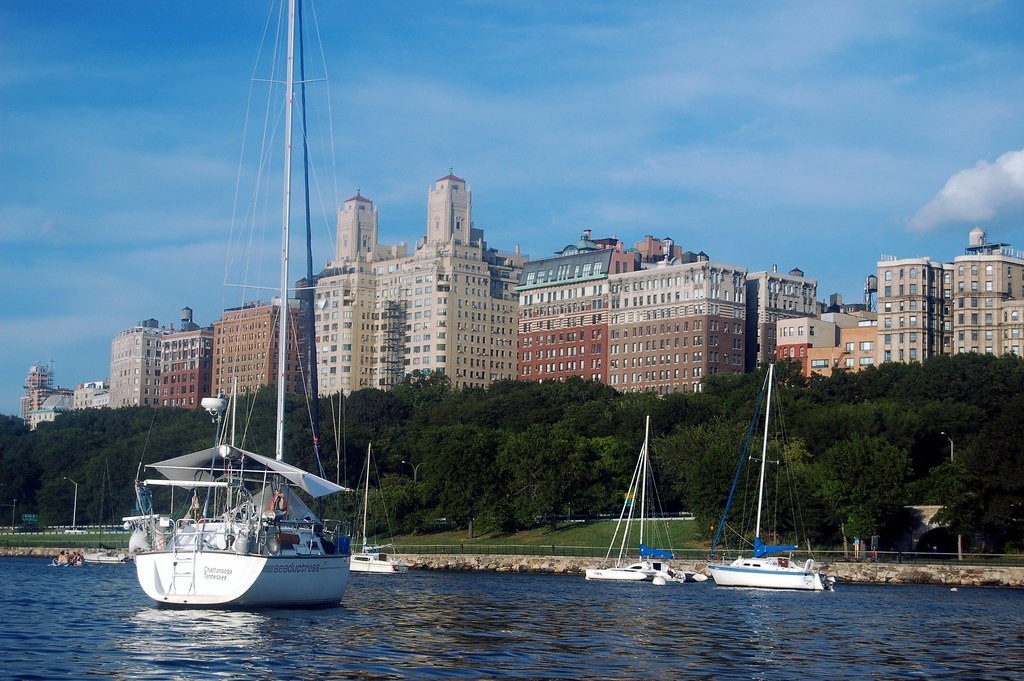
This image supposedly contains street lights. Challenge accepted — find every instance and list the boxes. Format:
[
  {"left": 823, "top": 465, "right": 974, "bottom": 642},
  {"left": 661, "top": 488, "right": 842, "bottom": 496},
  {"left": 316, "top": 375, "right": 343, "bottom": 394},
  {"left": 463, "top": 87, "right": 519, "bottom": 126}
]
[
  {"left": 13, "top": 499, "right": 17, "bottom": 529},
  {"left": 941, "top": 432, "right": 963, "bottom": 561},
  {"left": 63, "top": 477, "right": 79, "bottom": 529},
  {"left": 401, "top": 460, "right": 428, "bottom": 486}
]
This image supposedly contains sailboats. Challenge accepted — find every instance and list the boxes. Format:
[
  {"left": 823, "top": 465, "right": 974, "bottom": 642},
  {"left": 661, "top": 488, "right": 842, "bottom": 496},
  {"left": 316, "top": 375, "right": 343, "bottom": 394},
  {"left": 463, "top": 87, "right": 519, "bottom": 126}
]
[
  {"left": 350, "top": 441, "right": 411, "bottom": 574},
  {"left": 581, "top": 414, "right": 706, "bottom": 584},
  {"left": 78, "top": 471, "right": 126, "bottom": 565},
  {"left": 703, "top": 364, "right": 825, "bottom": 595},
  {"left": 117, "top": 3, "right": 365, "bottom": 609}
]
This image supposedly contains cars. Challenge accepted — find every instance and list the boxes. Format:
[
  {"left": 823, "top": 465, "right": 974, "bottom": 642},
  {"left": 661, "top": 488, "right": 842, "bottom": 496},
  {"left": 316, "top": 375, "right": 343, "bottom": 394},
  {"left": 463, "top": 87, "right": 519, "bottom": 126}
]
[{"left": 12, "top": 523, "right": 46, "bottom": 534}]
